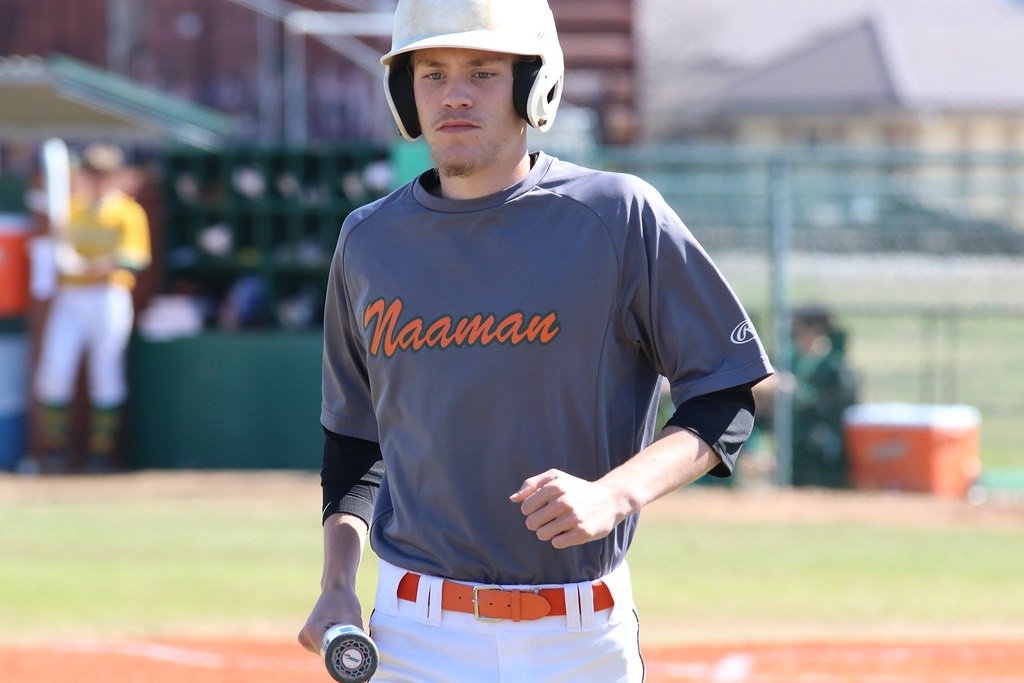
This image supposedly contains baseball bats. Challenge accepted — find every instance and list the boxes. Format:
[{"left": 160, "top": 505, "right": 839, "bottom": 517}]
[{"left": 317, "top": 620, "right": 378, "bottom": 683}]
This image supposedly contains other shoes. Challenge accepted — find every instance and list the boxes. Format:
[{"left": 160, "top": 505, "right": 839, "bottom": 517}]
[
  {"left": 14, "top": 452, "right": 72, "bottom": 474},
  {"left": 76, "top": 453, "right": 116, "bottom": 475}
]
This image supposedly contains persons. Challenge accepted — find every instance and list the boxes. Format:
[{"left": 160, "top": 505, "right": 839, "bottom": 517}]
[
  {"left": 298, "top": 0, "right": 774, "bottom": 683},
  {"left": 30, "top": 142, "right": 152, "bottom": 474},
  {"left": 751, "top": 299, "right": 865, "bottom": 491}
]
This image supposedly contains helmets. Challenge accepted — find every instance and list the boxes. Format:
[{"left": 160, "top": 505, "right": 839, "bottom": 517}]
[{"left": 379, "top": 0, "right": 565, "bottom": 142}]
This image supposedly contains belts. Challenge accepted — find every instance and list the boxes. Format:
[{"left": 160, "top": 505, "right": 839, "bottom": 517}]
[{"left": 397, "top": 572, "right": 614, "bottom": 622}]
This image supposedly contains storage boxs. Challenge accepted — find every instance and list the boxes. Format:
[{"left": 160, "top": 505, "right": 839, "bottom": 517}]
[{"left": 842, "top": 402, "right": 984, "bottom": 498}]
